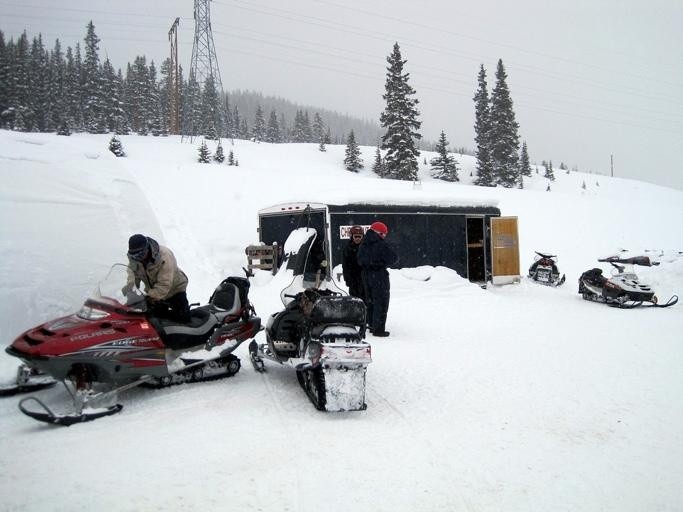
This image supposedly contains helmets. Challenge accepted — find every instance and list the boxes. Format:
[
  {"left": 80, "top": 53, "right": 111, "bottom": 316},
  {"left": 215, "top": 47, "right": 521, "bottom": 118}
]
[
  {"left": 370, "top": 221, "right": 387, "bottom": 234},
  {"left": 350, "top": 226, "right": 363, "bottom": 234}
]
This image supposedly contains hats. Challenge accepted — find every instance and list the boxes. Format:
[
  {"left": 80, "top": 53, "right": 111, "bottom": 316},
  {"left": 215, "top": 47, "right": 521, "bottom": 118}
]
[{"left": 127, "top": 234, "right": 146, "bottom": 255}]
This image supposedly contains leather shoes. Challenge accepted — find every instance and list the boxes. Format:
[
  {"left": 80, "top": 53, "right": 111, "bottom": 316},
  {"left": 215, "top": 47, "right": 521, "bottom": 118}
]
[{"left": 373, "top": 331, "right": 388, "bottom": 336}]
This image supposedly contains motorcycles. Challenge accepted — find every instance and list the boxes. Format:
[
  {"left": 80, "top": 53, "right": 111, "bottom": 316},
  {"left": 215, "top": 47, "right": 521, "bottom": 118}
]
[
  {"left": 2, "top": 263, "right": 261, "bottom": 426},
  {"left": 528, "top": 247, "right": 566, "bottom": 288},
  {"left": 576, "top": 261, "right": 678, "bottom": 310},
  {"left": 248, "top": 273, "right": 370, "bottom": 412}
]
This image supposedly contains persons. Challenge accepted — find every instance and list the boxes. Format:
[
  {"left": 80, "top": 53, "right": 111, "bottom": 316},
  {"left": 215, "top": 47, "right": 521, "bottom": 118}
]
[
  {"left": 120, "top": 234, "right": 190, "bottom": 325},
  {"left": 341, "top": 224, "right": 368, "bottom": 326},
  {"left": 356, "top": 221, "right": 400, "bottom": 337}
]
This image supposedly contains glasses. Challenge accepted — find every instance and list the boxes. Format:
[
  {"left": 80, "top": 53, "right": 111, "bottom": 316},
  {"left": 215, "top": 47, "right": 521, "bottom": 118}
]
[{"left": 126, "top": 248, "right": 147, "bottom": 261}]
